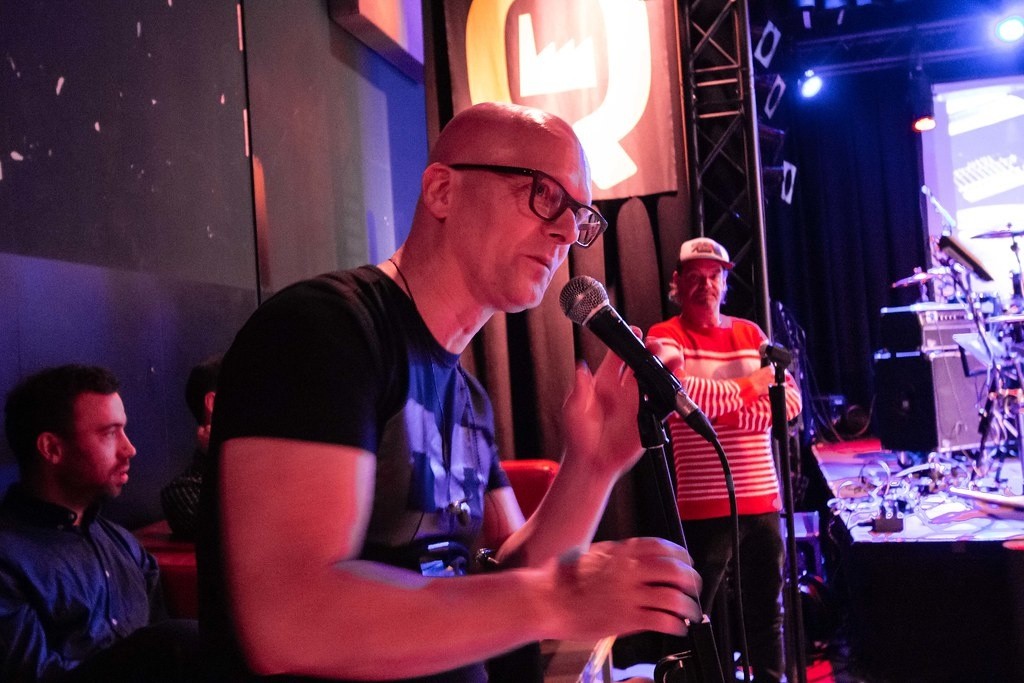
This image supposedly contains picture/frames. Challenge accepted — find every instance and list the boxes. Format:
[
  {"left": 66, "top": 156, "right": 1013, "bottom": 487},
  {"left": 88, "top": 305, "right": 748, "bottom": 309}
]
[{"left": 326, "top": 0, "right": 424, "bottom": 84}]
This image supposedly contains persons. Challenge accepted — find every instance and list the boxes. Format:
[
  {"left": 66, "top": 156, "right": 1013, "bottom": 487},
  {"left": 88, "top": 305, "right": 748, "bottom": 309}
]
[
  {"left": 645, "top": 236, "right": 804, "bottom": 683},
  {"left": 200, "top": 102, "right": 703, "bottom": 683},
  {"left": 0, "top": 355, "right": 234, "bottom": 683}
]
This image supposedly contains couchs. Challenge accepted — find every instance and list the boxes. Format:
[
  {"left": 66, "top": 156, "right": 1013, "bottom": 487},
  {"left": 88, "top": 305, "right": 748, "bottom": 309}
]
[{"left": 132, "top": 456, "right": 558, "bottom": 620}]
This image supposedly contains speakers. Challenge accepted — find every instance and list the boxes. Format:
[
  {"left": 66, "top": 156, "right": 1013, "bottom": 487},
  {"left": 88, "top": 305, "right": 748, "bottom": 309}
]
[
  {"left": 779, "top": 511, "right": 831, "bottom": 644},
  {"left": 874, "top": 344, "right": 1014, "bottom": 455}
]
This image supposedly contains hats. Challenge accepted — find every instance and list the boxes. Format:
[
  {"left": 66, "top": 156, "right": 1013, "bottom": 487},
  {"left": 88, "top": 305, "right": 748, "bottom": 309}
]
[{"left": 680, "top": 237, "right": 735, "bottom": 269}]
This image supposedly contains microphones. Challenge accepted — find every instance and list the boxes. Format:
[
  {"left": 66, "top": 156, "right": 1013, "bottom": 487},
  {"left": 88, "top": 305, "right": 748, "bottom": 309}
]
[
  {"left": 759, "top": 342, "right": 792, "bottom": 364},
  {"left": 559, "top": 275, "right": 718, "bottom": 442}
]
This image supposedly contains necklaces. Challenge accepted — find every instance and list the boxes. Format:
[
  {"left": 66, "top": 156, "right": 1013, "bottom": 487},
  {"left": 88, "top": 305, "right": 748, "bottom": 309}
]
[{"left": 389, "top": 257, "right": 416, "bottom": 302}]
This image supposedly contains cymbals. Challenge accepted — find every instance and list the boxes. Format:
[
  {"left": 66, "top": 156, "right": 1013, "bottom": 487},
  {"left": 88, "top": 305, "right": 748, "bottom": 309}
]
[
  {"left": 891, "top": 271, "right": 936, "bottom": 288},
  {"left": 969, "top": 228, "right": 1024, "bottom": 239},
  {"left": 980, "top": 313, "right": 1024, "bottom": 323}
]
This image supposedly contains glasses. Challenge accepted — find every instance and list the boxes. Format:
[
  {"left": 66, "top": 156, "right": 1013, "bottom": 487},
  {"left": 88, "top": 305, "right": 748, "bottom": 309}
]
[{"left": 449, "top": 163, "right": 609, "bottom": 249}]
